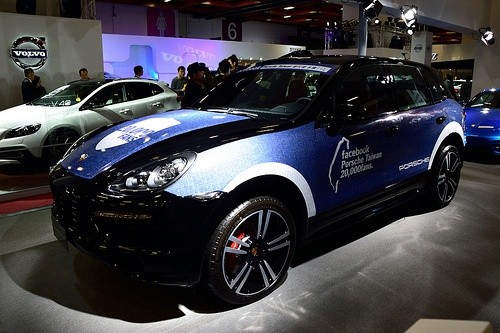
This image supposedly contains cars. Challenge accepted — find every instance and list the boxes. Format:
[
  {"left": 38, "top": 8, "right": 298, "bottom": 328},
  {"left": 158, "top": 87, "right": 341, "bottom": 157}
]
[
  {"left": 1, "top": 76, "right": 184, "bottom": 178},
  {"left": 461, "top": 87, "right": 500, "bottom": 164}
]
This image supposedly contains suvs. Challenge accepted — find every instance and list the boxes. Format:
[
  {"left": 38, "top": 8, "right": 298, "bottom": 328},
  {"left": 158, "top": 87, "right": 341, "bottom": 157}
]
[{"left": 45, "top": 53, "right": 468, "bottom": 307}]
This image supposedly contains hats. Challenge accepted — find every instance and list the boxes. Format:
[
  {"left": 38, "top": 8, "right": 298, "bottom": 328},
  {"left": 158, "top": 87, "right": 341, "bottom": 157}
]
[{"left": 187, "top": 62, "right": 207, "bottom": 74}]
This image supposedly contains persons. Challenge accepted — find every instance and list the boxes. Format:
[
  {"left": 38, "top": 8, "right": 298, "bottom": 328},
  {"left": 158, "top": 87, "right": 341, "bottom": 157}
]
[
  {"left": 185, "top": 54, "right": 244, "bottom": 106},
  {"left": 134, "top": 65, "right": 143, "bottom": 78},
  {"left": 444, "top": 74, "right": 472, "bottom": 102},
  {"left": 171, "top": 66, "right": 189, "bottom": 96},
  {"left": 288, "top": 71, "right": 311, "bottom": 96},
  {"left": 21, "top": 68, "right": 47, "bottom": 104},
  {"left": 79, "top": 68, "right": 90, "bottom": 79}
]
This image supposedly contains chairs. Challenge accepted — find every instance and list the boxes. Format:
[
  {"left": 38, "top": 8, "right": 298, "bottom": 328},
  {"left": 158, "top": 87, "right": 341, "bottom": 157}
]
[{"left": 285, "top": 78, "right": 307, "bottom": 103}]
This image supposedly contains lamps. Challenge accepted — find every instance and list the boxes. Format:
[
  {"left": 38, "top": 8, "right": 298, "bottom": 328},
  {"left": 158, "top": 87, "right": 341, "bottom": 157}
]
[
  {"left": 478, "top": 26, "right": 496, "bottom": 47},
  {"left": 363, "top": 0, "right": 384, "bottom": 22},
  {"left": 399, "top": 4, "right": 418, "bottom": 28}
]
